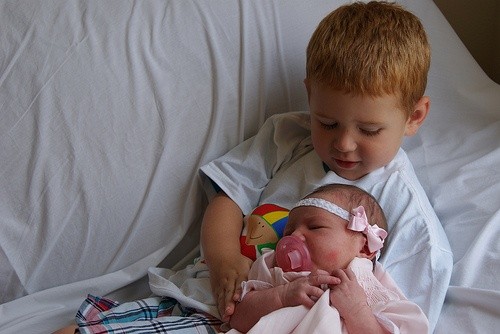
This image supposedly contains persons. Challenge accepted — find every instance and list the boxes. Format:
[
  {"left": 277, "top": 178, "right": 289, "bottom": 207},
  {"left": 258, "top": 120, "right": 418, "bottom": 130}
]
[
  {"left": 226, "top": 183, "right": 431, "bottom": 334},
  {"left": 50, "top": 0, "right": 453, "bottom": 334}
]
